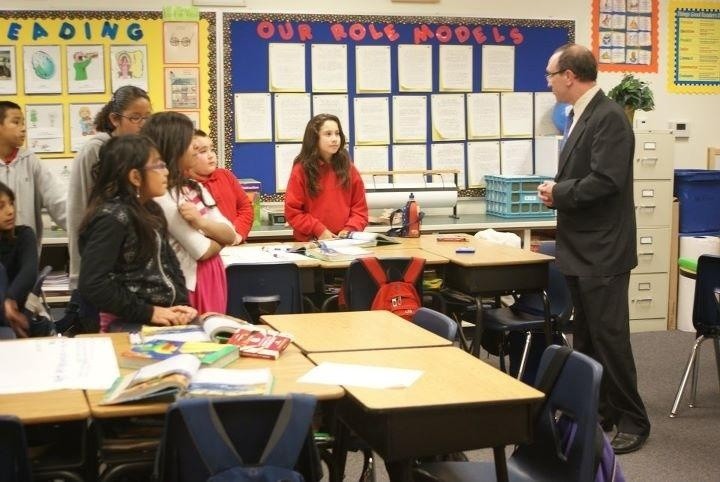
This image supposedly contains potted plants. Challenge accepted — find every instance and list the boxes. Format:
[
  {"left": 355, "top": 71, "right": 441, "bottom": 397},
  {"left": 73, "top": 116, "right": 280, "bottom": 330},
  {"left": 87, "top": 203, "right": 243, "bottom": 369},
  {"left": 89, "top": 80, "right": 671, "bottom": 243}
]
[{"left": 606, "top": 74, "right": 655, "bottom": 129}]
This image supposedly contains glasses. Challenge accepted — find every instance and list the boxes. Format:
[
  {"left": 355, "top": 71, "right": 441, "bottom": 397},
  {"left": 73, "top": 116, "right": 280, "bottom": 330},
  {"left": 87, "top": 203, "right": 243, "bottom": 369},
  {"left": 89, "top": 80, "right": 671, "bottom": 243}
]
[
  {"left": 544, "top": 69, "right": 565, "bottom": 79},
  {"left": 139, "top": 161, "right": 166, "bottom": 170},
  {"left": 114, "top": 112, "right": 149, "bottom": 124}
]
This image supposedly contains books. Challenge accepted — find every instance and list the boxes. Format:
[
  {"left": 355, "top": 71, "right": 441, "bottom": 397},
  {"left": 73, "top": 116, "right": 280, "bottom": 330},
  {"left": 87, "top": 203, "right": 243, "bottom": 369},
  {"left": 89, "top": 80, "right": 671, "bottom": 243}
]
[
  {"left": 286, "top": 232, "right": 401, "bottom": 261},
  {"left": 98, "top": 311, "right": 294, "bottom": 406}
]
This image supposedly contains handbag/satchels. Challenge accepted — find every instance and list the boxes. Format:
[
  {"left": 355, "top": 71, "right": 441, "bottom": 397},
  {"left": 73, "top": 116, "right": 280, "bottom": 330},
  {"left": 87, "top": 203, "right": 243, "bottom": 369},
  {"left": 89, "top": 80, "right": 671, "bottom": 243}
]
[
  {"left": 25, "top": 289, "right": 57, "bottom": 336},
  {"left": 421, "top": 274, "right": 443, "bottom": 289},
  {"left": 66, "top": 289, "right": 101, "bottom": 333}
]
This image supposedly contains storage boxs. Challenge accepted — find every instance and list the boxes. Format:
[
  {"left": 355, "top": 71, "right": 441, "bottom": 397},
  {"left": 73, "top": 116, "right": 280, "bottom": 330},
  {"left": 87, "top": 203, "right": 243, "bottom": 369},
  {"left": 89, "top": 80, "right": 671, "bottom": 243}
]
[{"left": 675, "top": 168, "right": 720, "bottom": 233}]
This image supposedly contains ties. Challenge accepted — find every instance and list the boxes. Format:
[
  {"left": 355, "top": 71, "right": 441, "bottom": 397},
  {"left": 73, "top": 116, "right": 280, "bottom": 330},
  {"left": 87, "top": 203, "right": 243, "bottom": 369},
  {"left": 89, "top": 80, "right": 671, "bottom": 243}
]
[{"left": 560, "top": 109, "right": 574, "bottom": 153}]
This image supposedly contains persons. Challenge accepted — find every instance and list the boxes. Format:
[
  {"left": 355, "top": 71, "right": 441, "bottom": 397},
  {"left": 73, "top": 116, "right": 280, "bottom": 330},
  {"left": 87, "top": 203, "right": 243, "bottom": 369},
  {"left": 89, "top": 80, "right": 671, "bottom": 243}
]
[
  {"left": 536, "top": 44, "right": 650, "bottom": 454},
  {"left": 284, "top": 113, "right": 369, "bottom": 242},
  {"left": 1, "top": 85, "right": 254, "bottom": 337}
]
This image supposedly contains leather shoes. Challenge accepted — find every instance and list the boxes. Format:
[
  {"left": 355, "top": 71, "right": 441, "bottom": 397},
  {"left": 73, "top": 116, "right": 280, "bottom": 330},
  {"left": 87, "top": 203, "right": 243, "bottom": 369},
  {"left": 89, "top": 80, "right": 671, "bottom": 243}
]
[
  {"left": 599, "top": 414, "right": 613, "bottom": 432},
  {"left": 611, "top": 432, "right": 649, "bottom": 452}
]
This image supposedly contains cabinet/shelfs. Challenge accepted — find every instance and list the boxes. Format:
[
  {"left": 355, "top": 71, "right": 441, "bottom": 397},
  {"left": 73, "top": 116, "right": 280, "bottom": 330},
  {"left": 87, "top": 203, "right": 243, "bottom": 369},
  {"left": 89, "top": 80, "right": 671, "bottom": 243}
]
[{"left": 628, "top": 129, "right": 676, "bottom": 333}]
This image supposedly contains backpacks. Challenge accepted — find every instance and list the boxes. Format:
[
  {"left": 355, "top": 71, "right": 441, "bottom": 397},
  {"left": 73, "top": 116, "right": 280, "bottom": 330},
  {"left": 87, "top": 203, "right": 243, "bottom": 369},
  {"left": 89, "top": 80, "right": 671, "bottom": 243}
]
[
  {"left": 440, "top": 282, "right": 523, "bottom": 316},
  {"left": 356, "top": 257, "right": 426, "bottom": 322},
  {"left": 538, "top": 346, "right": 624, "bottom": 482},
  {"left": 178, "top": 393, "right": 317, "bottom": 482}
]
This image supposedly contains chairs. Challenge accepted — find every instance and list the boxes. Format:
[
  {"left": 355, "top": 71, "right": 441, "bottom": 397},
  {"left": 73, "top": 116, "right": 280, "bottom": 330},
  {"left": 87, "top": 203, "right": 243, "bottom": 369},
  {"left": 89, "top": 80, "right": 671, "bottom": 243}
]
[
  {"left": 461, "top": 237, "right": 574, "bottom": 382},
  {"left": 414, "top": 344, "right": 604, "bottom": 481},
  {"left": 669, "top": 253, "right": 720, "bottom": 419}
]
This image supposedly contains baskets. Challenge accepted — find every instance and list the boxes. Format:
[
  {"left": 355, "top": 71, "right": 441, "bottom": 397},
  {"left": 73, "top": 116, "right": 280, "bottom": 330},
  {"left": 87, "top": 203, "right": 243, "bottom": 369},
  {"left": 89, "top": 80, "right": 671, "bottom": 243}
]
[{"left": 485, "top": 175, "right": 555, "bottom": 219}]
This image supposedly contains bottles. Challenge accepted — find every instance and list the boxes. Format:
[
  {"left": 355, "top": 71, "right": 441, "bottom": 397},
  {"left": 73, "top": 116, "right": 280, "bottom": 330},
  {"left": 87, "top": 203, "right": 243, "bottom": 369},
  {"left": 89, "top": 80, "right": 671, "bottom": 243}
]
[{"left": 401, "top": 192, "right": 421, "bottom": 237}]
